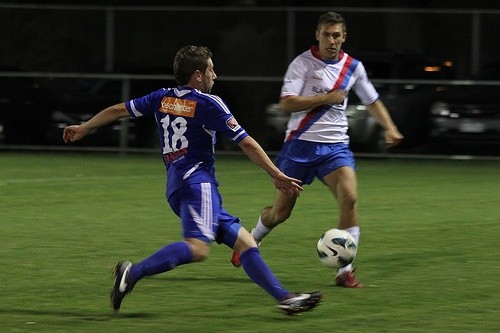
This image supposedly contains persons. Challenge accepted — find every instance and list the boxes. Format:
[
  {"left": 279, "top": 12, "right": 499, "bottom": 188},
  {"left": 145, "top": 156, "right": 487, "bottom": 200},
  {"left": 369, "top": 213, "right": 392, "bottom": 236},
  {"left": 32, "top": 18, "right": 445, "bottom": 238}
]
[
  {"left": 61, "top": 44, "right": 326, "bottom": 318},
  {"left": 231, "top": 10, "right": 403, "bottom": 288}
]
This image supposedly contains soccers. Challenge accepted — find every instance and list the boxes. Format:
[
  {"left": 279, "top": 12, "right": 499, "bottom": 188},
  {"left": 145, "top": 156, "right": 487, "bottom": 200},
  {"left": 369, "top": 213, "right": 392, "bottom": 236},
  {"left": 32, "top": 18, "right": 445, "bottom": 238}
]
[{"left": 316, "top": 228, "right": 357, "bottom": 268}]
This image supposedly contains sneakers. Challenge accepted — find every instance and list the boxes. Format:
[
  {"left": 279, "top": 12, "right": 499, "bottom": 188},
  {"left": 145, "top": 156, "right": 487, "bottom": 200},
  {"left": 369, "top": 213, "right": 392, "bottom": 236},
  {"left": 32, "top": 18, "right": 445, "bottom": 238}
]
[
  {"left": 231, "top": 229, "right": 261, "bottom": 268},
  {"left": 277, "top": 291, "right": 324, "bottom": 316},
  {"left": 110, "top": 260, "right": 136, "bottom": 311},
  {"left": 335, "top": 267, "right": 364, "bottom": 288}
]
